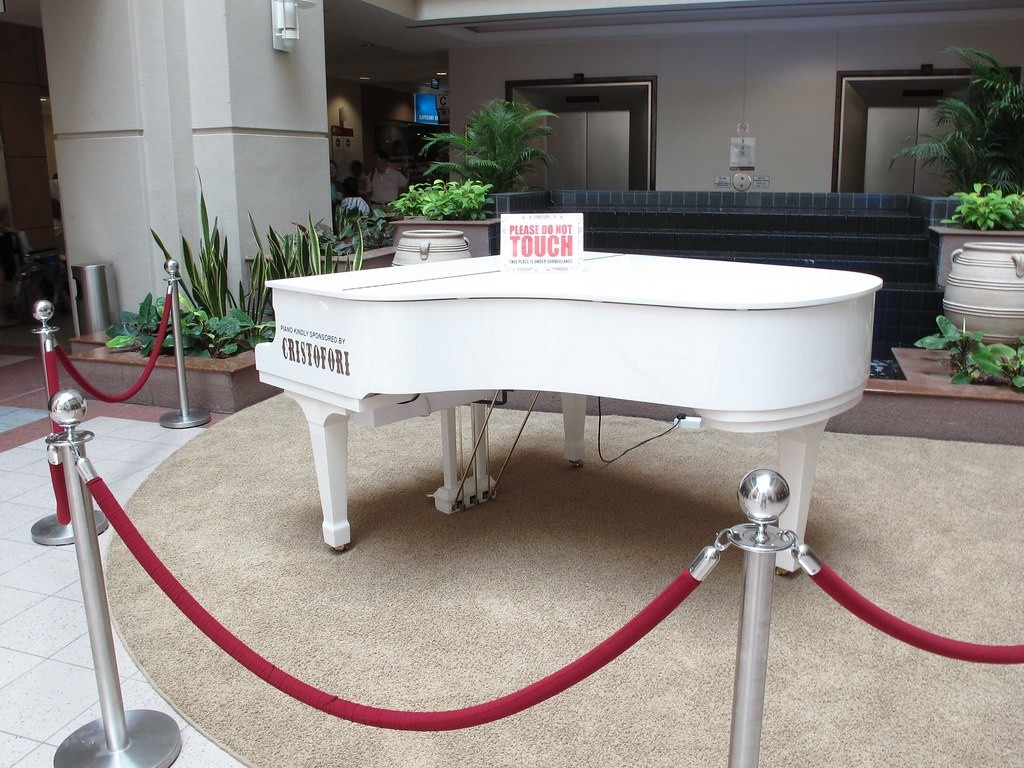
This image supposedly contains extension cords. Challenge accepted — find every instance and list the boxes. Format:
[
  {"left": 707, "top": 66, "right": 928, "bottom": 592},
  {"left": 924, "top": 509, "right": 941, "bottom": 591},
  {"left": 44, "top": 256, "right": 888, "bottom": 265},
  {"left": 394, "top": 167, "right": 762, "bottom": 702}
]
[{"left": 674, "top": 417, "right": 703, "bottom": 429}]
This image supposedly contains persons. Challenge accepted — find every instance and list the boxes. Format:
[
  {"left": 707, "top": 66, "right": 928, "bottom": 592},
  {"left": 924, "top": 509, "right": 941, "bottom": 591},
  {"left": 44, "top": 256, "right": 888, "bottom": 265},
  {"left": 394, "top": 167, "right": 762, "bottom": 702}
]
[
  {"left": 0, "top": 203, "right": 25, "bottom": 283},
  {"left": 49, "top": 173, "right": 63, "bottom": 230},
  {"left": 330, "top": 149, "right": 449, "bottom": 251}
]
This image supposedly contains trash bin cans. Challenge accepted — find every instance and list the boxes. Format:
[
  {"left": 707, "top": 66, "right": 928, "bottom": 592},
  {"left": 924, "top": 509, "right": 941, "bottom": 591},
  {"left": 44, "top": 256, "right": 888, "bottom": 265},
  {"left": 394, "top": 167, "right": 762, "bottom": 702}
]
[{"left": 70, "top": 262, "right": 118, "bottom": 335}]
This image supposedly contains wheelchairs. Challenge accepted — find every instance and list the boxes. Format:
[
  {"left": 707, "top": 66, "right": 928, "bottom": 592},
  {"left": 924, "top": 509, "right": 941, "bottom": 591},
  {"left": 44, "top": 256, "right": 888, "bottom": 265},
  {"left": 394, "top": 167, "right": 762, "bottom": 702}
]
[{"left": 0, "top": 231, "right": 69, "bottom": 325}]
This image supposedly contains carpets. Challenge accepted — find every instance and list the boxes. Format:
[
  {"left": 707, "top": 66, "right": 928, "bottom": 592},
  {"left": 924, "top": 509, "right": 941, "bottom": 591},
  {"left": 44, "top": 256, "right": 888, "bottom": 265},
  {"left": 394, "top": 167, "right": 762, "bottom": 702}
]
[{"left": 106, "top": 388, "right": 1024, "bottom": 768}]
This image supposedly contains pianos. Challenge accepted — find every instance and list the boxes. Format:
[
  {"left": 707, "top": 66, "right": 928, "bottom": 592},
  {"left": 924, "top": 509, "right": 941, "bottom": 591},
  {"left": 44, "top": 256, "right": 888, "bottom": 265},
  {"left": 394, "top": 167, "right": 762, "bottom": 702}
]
[{"left": 253, "top": 248, "right": 885, "bottom": 580}]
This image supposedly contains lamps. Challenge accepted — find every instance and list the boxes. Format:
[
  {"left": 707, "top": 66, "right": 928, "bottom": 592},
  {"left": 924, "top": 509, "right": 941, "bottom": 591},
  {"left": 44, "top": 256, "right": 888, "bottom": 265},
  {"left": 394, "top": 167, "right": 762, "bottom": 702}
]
[
  {"left": 271, "top": 0, "right": 300, "bottom": 53},
  {"left": 339, "top": 106, "right": 348, "bottom": 122}
]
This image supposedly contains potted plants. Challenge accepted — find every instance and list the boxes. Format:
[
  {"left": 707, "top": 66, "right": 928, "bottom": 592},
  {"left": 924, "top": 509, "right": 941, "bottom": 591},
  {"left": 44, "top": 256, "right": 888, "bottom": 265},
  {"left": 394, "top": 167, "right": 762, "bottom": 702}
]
[
  {"left": 387, "top": 179, "right": 500, "bottom": 256},
  {"left": 927, "top": 184, "right": 1024, "bottom": 287}
]
